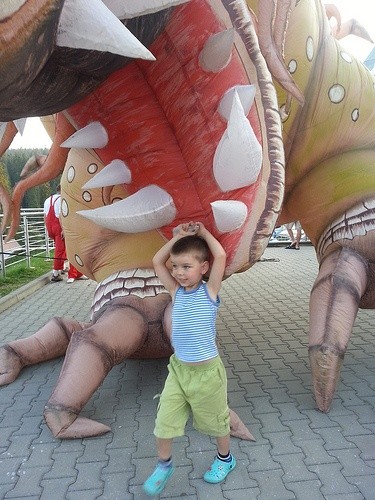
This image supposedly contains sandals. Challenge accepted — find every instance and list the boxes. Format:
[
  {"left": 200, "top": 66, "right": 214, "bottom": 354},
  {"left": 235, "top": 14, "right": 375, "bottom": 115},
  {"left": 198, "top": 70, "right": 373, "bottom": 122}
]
[
  {"left": 143, "top": 461, "right": 175, "bottom": 498},
  {"left": 202, "top": 453, "right": 237, "bottom": 485}
]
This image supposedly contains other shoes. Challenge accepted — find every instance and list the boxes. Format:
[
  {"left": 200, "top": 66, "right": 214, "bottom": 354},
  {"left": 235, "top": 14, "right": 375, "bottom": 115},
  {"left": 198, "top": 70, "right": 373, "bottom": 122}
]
[
  {"left": 75, "top": 274, "right": 89, "bottom": 280},
  {"left": 66, "top": 278, "right": 75, "bottom": 283},
  {"left": 51, "top": 273, "right": 64, "bottom": 281},
  {"left": 285, "top": 244, "right": 300, "bottom": 250}
]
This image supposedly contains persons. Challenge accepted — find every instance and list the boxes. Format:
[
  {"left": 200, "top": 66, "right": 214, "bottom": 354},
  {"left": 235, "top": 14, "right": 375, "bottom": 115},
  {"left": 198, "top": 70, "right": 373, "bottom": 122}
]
[
  {"left": 285, "top": 221, "right": 302, "bottom": 250},
  {"left": 66, "top": 263, "right": 89, "bottom": 283},
  {"left": 144, "top": 220, "right": 237, "bottom": 497},
  {"left": 43, "top": 184, "right": 67, "bottom": 281}
]
